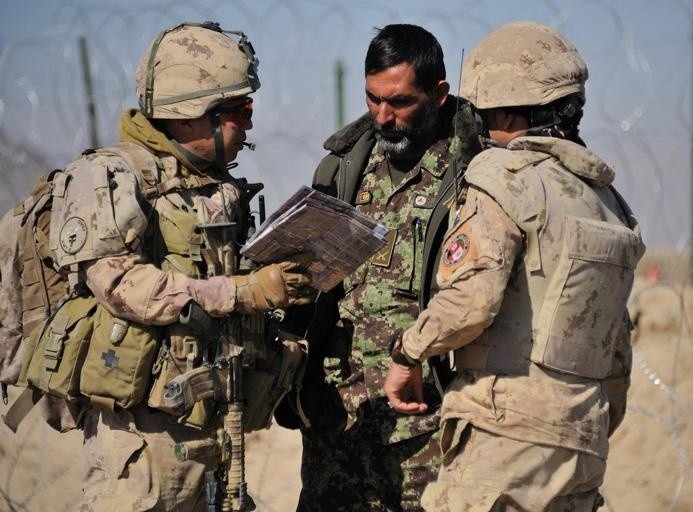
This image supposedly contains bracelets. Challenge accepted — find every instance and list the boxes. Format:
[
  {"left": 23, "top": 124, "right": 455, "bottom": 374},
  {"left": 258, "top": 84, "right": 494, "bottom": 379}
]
[{"left": 387, "top": 330, "right": 416, "bottom": 368}]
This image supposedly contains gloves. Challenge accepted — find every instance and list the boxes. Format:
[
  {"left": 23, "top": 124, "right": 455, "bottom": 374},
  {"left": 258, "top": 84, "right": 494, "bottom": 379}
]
[{"left": 228, "top": 251, "right": 315, "bottom": 315}]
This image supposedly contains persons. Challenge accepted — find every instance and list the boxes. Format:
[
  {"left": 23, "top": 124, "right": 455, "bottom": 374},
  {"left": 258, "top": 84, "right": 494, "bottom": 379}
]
[
  {"left": 1, "top": 20, "right": 319, "bottom": 510},
  {"left": 271, "top": 22, "right": 489, "bottom": 511},
  {"left": 381, "top": 19, "right": 650, "bottom": 512}
]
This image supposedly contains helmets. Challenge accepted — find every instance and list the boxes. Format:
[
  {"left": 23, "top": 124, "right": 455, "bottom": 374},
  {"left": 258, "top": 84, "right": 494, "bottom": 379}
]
[
  {"left": 453, "top": 19, "right": 588, "bottom": 110},
  {"left": 135, "top": 22, "right": 261, "bottom": 123}
]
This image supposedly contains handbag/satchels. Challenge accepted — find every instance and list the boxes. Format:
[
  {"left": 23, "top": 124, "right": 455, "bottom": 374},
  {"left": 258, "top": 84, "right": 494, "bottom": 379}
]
[
  {"left": 238, "top": 323, "right": 309, "bottom": 436},
  {"left": 25, "top": 291, "right": 160, "bottom": 416}
]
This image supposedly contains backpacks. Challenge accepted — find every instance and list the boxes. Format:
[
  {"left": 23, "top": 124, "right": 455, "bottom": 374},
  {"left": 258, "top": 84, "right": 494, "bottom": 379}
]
[{"left": 0, "top": 140, "right": 162, "bottom": 391}]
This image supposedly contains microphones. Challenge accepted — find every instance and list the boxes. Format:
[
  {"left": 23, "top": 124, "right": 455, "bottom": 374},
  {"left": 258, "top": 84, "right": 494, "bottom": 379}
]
[{"left": 243, "top": 141, "right": 256, "bottom": 151}]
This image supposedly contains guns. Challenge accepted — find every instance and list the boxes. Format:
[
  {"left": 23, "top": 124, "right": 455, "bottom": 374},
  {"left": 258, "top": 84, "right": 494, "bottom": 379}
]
[{"left": 166, "top": 227, "right": 266, "bottom": 512}]
